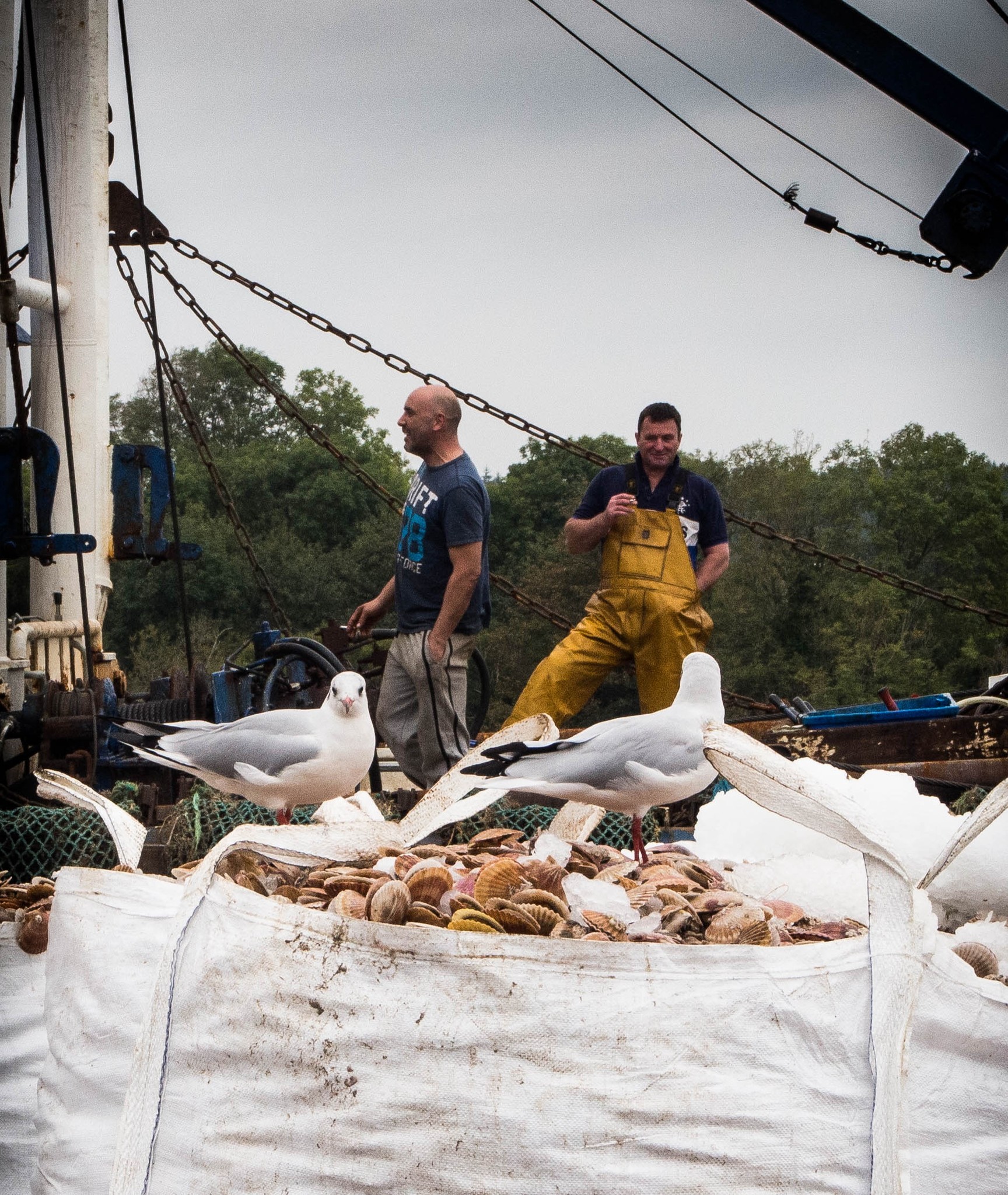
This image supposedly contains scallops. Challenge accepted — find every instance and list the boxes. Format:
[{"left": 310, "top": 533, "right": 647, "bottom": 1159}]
[{"left": 1, "top": 825, "right": 1006, "bottom": 987}]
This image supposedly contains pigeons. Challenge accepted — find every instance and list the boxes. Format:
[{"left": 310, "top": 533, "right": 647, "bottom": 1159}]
[
  {"left": 465, "top": 652, "right": 722, "bottom": 865},
  {"left": 103, "top": 670, "right": 376, "bottom": 825}
]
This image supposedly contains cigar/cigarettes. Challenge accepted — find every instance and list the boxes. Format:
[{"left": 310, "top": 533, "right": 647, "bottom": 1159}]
[
  {"left": 629, "top": 498, "right": 634, "bottom": 504},
  {"left": 339, "top": 625, "right": 357, "bottom": 629}
]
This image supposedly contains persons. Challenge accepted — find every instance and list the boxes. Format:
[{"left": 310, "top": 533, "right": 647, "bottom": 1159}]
[
  {"left": 347, "top": 384, "right": 493, "bottom": 798},
  {"left": 500, "top": 402, "right": 733, "bottom": 734}
]
